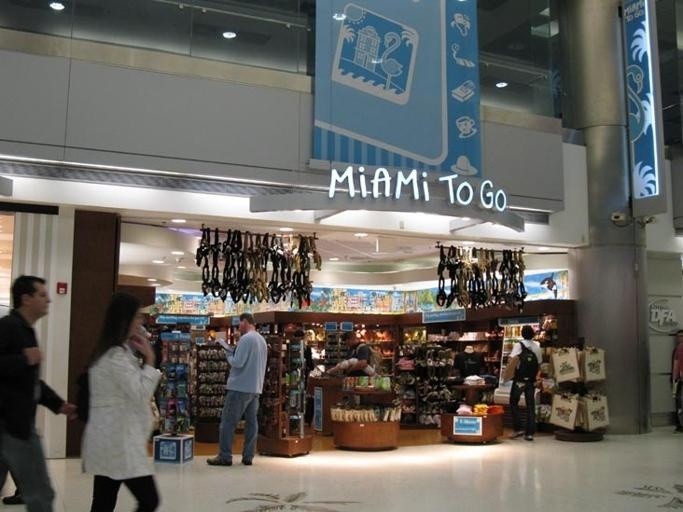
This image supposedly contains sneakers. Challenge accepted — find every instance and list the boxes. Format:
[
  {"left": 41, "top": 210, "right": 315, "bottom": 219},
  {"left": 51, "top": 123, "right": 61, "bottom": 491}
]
[
  {"left": 525, "top": 435, "right": 533, "bottom": 440},
  {"left": 207, "top": 455, "right": 232, "bottom": 465},
  {"left": 242, "top": 460, "right": 252, "bottom": 465},
  {"left": 508, "top": 431, "right": 525, "bottom": 438}
]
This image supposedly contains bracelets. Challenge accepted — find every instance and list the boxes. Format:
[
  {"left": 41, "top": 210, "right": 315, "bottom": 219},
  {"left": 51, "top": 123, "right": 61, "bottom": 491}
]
[{"left": 672, "top": 378, "right": 676, "bottom": 382}]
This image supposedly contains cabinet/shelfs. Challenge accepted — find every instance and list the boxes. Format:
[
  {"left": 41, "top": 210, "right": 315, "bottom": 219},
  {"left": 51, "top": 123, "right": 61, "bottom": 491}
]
[
  {"left": 223, "top": 324, "right": 280, "bottom": 348},
  {"left": 429, "top": 315, "right": 551, "bottom": 376},
  {"left": 196, "top": 345, "right": 225, "bottom": 423},
  {"left": 329, "top": 386, "right": 400, "bottom": 452},
  {"left": 441, "top": 383, "right": 501, "bottom": 445},
  {"left": 155, "top": 338, "right": 194, "bottom": 437},
  {"left": 255, "top": 351, "right": 310, "bottom": 457},
  {"left": 400, "top": 345, "right": 452, "bottom": 429},
  {"left": 305, "top": 323, "right": 397, "bottom": 374}
]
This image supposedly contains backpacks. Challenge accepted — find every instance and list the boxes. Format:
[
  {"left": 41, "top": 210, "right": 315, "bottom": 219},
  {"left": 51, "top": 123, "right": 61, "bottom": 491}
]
[{"left": 514, "top": 341, "right": 538, "bottom": 385}]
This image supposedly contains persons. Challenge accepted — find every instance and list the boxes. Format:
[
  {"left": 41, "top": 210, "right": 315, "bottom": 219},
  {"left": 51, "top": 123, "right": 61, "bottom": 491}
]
[
  {"left": 75, "top": 288, "right": 166, "bottom": 510},
  {"left": 0, "top": 272, "right": 79, "bottom": 510},
  {"left": 502, "top": 322, "right": 544, "bottom": 444},
  {"left": 200, "top": 311, "right": 263, "bottom": 471},
  {"left": 339, "top": 330, "right": 372, "bottom": 376},
  {"left": 670, "top": 329, "right": 682, "bottom": 433}
]
